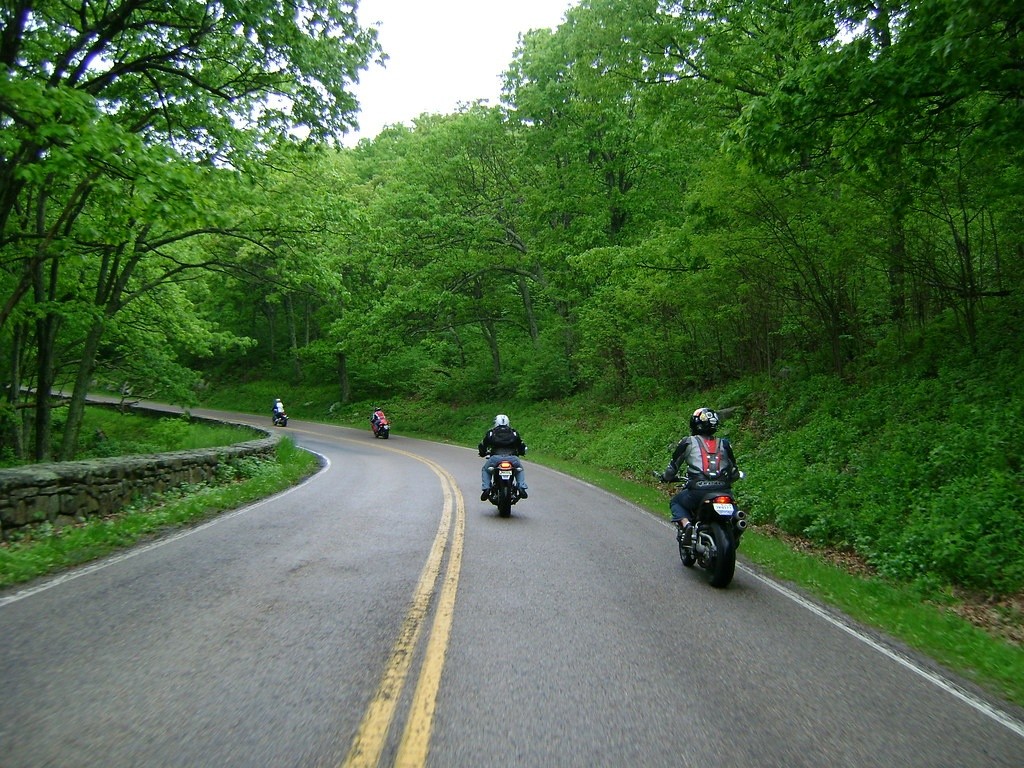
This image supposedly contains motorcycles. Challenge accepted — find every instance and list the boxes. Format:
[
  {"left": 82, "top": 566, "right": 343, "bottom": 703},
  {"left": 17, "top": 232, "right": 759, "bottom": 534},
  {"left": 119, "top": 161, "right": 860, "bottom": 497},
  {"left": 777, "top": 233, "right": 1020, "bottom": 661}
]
[
  {"left": 272, "top": 409, "right": 290, "bottom": 427},
  {"left": 370, "top": 418, "right": 390, "bottom": 439},
  {"left": 478, "top": 446, "right": 532, "bottom": 519},
  {"left": 653, "top": 469, "right": 748, "bottom": 589}
]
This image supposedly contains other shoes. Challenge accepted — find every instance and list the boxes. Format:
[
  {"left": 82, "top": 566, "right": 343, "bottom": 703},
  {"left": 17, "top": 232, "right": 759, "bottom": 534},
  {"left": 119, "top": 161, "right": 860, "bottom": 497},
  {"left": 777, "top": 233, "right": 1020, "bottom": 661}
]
[
  {"left": 519, "top": 488, "right": 527, "bottom": 499},
  {"left": 375, "top": 430, "right": 379, "bottom": 435},
  {"left": 275, "top": 419, "right": 279, "bottom": 423},
  {"left": 481, "top": 489, "right": 490, "bottom": 501},
  {"left": 680, "top": 523, "right": 693, "bottom": 550}
]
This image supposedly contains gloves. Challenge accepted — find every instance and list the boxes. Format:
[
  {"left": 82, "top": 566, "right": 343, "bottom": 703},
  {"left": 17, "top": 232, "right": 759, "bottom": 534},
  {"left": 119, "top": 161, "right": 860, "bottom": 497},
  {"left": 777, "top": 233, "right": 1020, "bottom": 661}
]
[{"left": 659, "top": 474, "right": 672, "bottom": 484}]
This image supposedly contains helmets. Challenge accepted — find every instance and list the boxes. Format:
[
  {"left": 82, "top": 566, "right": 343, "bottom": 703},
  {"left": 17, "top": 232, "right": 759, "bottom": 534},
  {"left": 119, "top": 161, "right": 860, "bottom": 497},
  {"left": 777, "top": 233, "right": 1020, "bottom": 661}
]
[
  {"left": 374, "top": 407, "right": 381, "bottom": 412},
  {"left": 690, "top": 408, "right": 719, "bottom": 436},
  {"left": 275, "top": 399, "right": 281, "bottom": 403},
  {"left": 494, "top": 414, "right": 509, "bottom": 428}
]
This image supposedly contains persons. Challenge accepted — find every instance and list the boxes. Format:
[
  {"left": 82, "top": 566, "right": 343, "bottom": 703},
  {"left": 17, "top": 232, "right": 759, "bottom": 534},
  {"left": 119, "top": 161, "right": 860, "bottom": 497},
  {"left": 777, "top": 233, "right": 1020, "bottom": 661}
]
[
  {"left": 271, "top": 398, "right": 284, "bottom": 425},
  {"left": 370, "top": 407, "right": 387, "bottom": 436},
  {"left": 659, "top": 408, "right": 741, "bottom": 548},
  {"left": 478, "top": 414, "right": 528, "bottom": 502}
]
